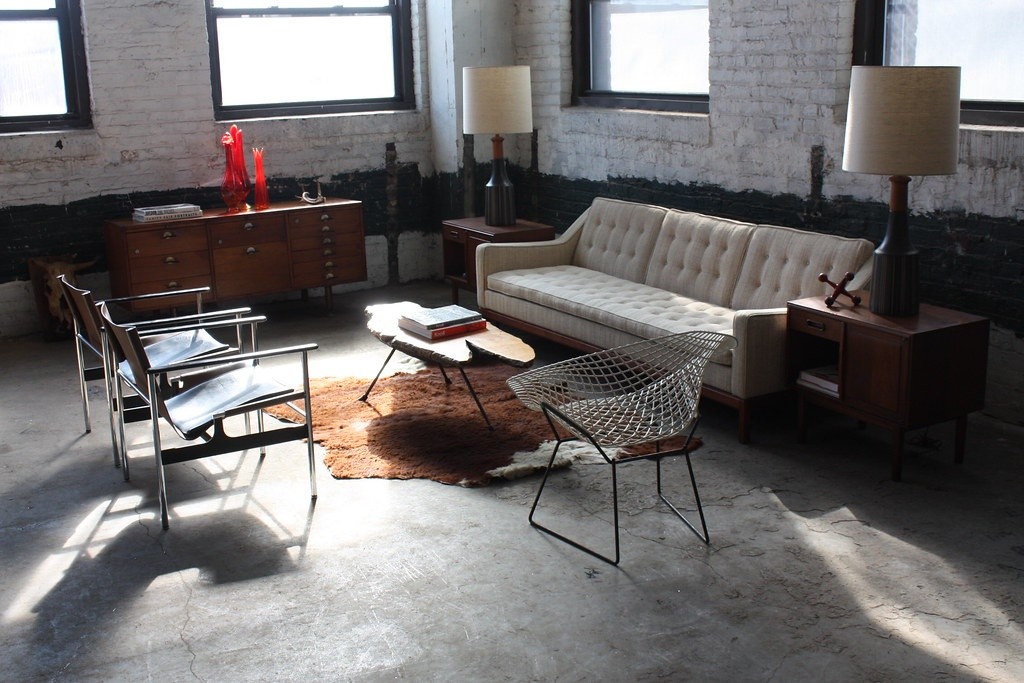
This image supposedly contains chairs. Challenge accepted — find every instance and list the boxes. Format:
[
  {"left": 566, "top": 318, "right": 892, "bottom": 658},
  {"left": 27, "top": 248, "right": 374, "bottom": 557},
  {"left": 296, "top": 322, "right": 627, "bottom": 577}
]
[
  {"left": 94, "top": 300, "right": 319, "bottom": 532},
  {"left": 55, "top": 274, "right": 252, "bottom": 468},
  {"left": 507, "top": 331, "right": 740, "bottom": 565}
]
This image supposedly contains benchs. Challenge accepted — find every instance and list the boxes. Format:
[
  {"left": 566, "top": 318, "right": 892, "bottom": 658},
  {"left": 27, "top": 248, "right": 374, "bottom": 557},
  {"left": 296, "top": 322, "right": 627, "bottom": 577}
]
[{"left": 475, "top": 196, "right": 875, "bottom": 439}]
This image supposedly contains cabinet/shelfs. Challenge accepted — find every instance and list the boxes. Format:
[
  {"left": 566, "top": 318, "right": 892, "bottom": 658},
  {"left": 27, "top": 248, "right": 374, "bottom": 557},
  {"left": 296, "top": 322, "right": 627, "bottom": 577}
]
[{"left": 105, "top": 197, "right": 367, "bottom": 322}]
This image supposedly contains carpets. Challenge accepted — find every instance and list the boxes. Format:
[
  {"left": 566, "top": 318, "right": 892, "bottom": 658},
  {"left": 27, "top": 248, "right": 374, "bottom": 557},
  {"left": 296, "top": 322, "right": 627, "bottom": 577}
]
[{"left": 265, "top": 349, "right": 703, "bottom": 491}]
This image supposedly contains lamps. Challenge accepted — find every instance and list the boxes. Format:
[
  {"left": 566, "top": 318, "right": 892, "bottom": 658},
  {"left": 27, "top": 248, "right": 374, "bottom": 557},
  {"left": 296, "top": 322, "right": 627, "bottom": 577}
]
[
  {"left": 841, "top": 65, "right": 961, "bottom": 312},
  {"left": 462, "top": 66, "right": 532, "bottom": 227}
]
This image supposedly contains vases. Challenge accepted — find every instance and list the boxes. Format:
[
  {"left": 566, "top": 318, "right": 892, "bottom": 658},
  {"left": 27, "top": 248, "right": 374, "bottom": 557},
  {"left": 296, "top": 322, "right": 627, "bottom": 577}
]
[
  {"left": 252, "top": 147, "right": 269, "bottom": 210},
  {"left": 222, "top": 132, "right": 245, "bottom": 213},
  {"left": 228, "top": 125, "right": 251, "bottom": 210}
]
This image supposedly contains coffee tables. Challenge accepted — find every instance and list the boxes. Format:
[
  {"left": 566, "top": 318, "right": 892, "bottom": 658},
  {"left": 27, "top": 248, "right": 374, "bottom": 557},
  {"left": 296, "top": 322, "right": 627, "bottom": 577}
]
[{"left": 357, "top": 301, "right": 535, "bottom": 437}]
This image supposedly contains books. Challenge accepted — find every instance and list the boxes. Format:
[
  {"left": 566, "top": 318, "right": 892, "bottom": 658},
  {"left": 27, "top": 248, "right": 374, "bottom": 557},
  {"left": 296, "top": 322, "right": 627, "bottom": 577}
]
[
  {"left": 794, "top": 364, "right": 838, "bottom": 399},
  {"left": 399, "top": 303, "right": 487, "bottom": 339},
  {"left": 130, "top": 204, "right": 205, "bottom": 221}
]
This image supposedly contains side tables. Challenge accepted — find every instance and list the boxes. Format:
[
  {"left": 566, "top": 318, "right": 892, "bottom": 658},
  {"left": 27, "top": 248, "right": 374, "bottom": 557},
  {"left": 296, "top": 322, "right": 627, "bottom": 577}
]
[
  {"left": 442, "top": 217, "right": 554, "bottom": 306},
  {"left": 783, "top": 291, "right": 988, "bottom": 483}
]
